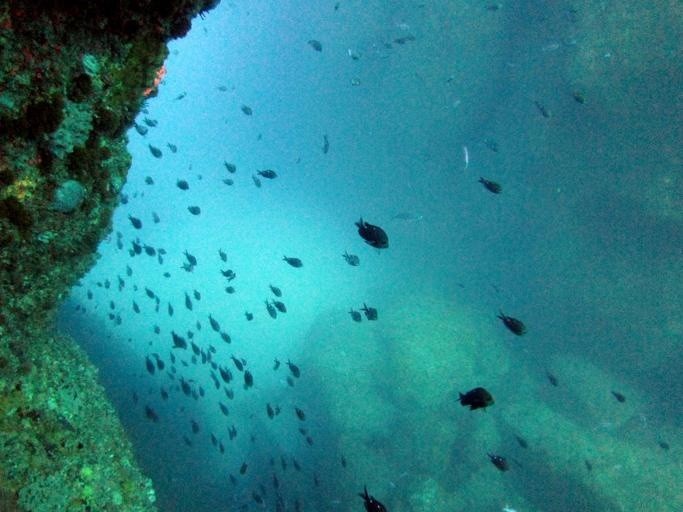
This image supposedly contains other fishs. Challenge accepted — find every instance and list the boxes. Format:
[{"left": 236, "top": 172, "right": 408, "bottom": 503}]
[{"left": 0, "top": 0, "right": 423, "bottom": 511}]
